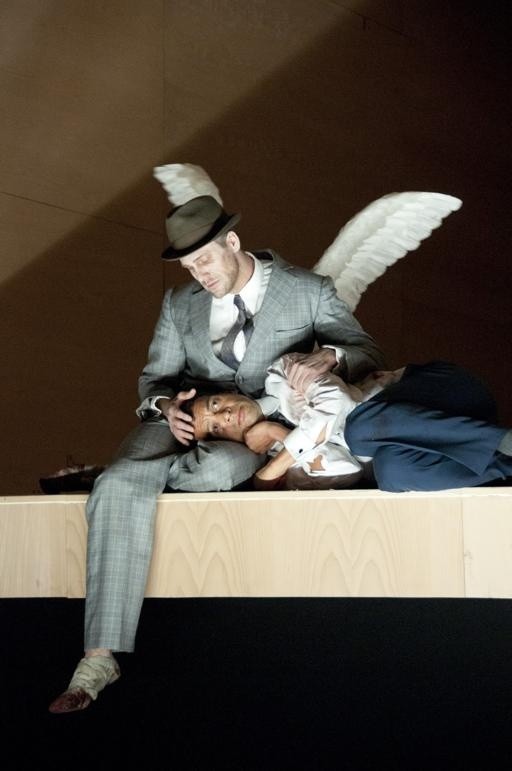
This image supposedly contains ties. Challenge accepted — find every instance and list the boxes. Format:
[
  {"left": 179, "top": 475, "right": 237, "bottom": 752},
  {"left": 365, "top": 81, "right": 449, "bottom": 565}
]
[{"left": 221, "top": 294, "right": 248, "bottom": 371}]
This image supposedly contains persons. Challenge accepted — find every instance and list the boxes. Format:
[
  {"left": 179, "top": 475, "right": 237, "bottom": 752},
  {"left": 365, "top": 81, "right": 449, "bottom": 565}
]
[
  {"left": 180, "top": 351, "right": 512, "bottom": 493},
  {"left": 48, "top": 194, "right": 385, "bottom": 713}
]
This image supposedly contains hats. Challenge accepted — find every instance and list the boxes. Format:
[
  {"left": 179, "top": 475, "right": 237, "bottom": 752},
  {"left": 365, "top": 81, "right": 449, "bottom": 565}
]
[{"left": 160, "top": 195, "right": 241, "bottom": 263}]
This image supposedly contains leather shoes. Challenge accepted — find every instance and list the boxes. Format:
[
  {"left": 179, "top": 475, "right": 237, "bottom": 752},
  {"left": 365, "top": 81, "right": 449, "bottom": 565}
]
[{"left": 48, "top": 656, "right": 121, "bottom": 713}]
